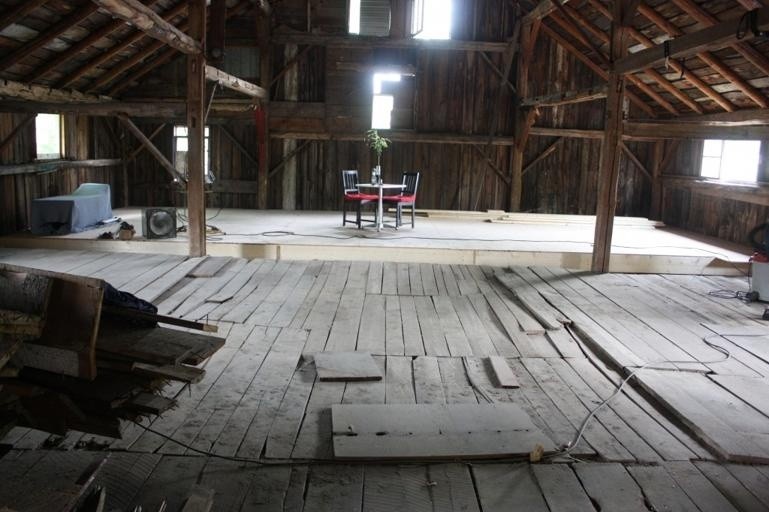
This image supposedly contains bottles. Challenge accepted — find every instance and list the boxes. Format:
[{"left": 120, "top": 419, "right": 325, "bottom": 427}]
[{"left": 371, "top": 169, "right": 378, "bottom": 185}]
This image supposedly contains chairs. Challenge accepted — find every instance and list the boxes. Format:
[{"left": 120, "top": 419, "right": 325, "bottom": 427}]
[
  {"left": 342, "top": 169, "right": 378, "bottom": 228},
  {"left": 383, "top": 171, "right": 419, "bottom": 230}
]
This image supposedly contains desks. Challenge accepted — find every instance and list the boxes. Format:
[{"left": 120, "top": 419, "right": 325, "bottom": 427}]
[{"left": 356, "top": 183, "right": 407, "bottom": 232}]
